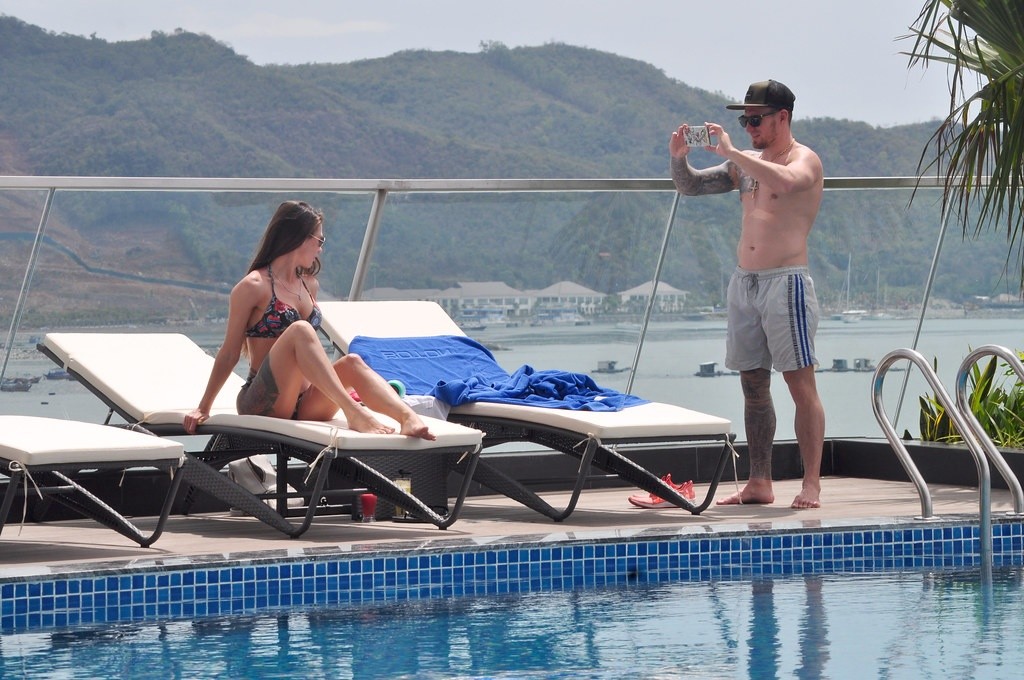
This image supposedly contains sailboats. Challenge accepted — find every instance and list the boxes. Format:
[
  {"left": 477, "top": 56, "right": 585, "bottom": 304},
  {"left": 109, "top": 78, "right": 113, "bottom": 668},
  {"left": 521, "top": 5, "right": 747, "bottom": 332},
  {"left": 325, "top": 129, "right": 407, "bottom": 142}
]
[{"left": 831, "top": 251, "right": 893, "bottom": 322}]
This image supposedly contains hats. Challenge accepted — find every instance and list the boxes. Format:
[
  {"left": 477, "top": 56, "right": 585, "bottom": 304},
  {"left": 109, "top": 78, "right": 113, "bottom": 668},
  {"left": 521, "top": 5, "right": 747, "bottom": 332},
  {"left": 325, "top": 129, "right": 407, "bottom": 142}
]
[{"left": 726, "top": 79, "right": 794, "bottom": 110}]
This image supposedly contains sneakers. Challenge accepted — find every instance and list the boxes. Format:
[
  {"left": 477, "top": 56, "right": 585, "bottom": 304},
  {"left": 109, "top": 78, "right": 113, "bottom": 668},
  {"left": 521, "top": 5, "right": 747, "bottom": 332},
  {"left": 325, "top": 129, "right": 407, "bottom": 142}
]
[{"left": 629, "top": 473, "right": 696, "bottom": 508}]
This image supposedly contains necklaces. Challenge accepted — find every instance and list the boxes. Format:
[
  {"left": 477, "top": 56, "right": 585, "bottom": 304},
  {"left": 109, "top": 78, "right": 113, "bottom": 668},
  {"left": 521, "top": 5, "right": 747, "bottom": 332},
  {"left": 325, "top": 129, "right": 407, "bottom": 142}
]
[
  {"left": 750, "top": 137, "right": 796, "bottom": 198},
  {"left": 272, "top": 270, "right": 302, "bottom": 300}
]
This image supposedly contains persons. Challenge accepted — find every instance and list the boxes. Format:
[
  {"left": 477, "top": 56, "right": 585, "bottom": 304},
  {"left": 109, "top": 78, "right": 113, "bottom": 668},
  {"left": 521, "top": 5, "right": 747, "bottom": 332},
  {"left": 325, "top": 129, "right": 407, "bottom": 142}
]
[
  {"left": 668, "top": 79, "right": 826, "bottom": 508},
  {"left": 183, "top": 200, "right": 436, "bottom": 442}
]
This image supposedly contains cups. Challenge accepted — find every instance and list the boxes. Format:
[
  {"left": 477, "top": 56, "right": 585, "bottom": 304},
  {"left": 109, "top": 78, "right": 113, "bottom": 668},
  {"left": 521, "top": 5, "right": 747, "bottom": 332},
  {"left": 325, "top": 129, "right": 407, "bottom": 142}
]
[
  {"left": 360, "top": 494, "right": 377, "bottom": 522},
  {"left": 392, "top": 478, "right": 411, "bottom": 515}
]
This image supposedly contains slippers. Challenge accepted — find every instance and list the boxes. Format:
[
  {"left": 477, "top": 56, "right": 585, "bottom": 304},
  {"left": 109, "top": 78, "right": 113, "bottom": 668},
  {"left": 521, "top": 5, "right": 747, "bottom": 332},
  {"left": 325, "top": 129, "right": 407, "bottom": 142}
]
[{"left": 391, "top": 505, "right": 450, "bottom": 522}]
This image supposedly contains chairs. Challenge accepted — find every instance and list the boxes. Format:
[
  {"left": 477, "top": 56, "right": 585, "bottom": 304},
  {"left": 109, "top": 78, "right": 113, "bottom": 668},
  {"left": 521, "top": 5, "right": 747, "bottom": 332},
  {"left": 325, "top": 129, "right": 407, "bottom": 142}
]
[
  {"left": 0, "top": 415, "right": 187, "bottom": 548},
  {"left": 36, "top": 332, "right": 483, "bottom": 539},
  {"left": 316, "top": 301, "right": 736, "bottom": 522}
]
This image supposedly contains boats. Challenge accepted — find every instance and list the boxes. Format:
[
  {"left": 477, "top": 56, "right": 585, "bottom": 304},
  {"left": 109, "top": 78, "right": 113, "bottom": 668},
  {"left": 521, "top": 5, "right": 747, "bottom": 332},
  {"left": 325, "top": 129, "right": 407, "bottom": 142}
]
[
  {"left": 0, "top": 369, "right": 77, "bottom": 392},
  {"left": 815, "top": 359, "right": 853, "bottom": 372},
  {"left": 694, "top": 362, "right": 740, "bottom": 377},
  {"left": 459, "top": 323, "right": 488, "bottom": 331},
  {"left": 852, "top": 358, "right": 905, "bottom": 371},
  {"left": 592, "top": 360, "right": 631, "bottom": 373}
]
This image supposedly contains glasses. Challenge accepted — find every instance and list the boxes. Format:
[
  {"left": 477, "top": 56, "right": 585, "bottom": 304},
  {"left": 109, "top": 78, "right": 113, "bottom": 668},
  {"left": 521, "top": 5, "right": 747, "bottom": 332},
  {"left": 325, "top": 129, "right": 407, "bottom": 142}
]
[
  {"left": 738, "top": 110, "right": 780, "bottom": 127},
  {"left": 309, "top": 234, "right": 326, "bottom": 247}
]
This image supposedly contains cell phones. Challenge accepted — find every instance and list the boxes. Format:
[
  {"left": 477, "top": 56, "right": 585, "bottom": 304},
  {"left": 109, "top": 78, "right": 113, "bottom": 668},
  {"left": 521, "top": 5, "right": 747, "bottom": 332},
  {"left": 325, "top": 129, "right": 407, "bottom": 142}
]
[{"left": 683, "top": 126, "right": 711, "bottom": 148}]
